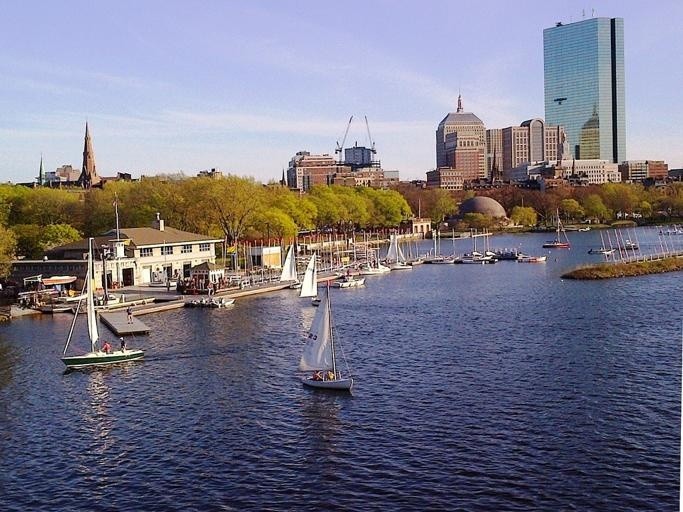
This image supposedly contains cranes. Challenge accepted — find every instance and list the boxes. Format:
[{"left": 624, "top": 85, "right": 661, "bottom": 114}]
[{"left": 335, "top": 114, "right": 378, "bottom": 164}]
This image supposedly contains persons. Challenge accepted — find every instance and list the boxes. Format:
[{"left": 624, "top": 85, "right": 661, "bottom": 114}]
[
  {"left": 166, "top": 279, "right": 170, "bottom": 291},
  {"left": 120, "top": 337, "right": 126, "bottom": 353},
  {"left": 103, "top": 341, "right": 110, "bottom": 354},
  {"left": 127, "top": 306, "right": 133, "bottom": 324},
  {"left": 313, "top": 371, "right": 335, "bottom": 381}
]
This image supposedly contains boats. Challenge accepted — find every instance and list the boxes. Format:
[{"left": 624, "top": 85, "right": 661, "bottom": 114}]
[
  {"left": 299, "top": 280, "right": 353, "bottom": 389},
  {"left": 61, "top": 237, "right": 144, "bottom": 370},
  {"left": 587, "top": 239, "right": 639, "bottom": 255},
  {"left": 279, "top": 208, "right": 569, "bottom": 306},
  {"left": 188, "top": 297, "right": 235, "bottom": 309}
]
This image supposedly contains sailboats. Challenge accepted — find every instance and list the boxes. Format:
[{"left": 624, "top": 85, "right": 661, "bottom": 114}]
[{"left": 656, "top": 224, "right": 683, "bottom": 236}]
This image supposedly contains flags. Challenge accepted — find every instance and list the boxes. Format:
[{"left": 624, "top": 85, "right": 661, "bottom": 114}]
[{"left": 226, "top": 246, "right": 236, "bottom": 255}]
